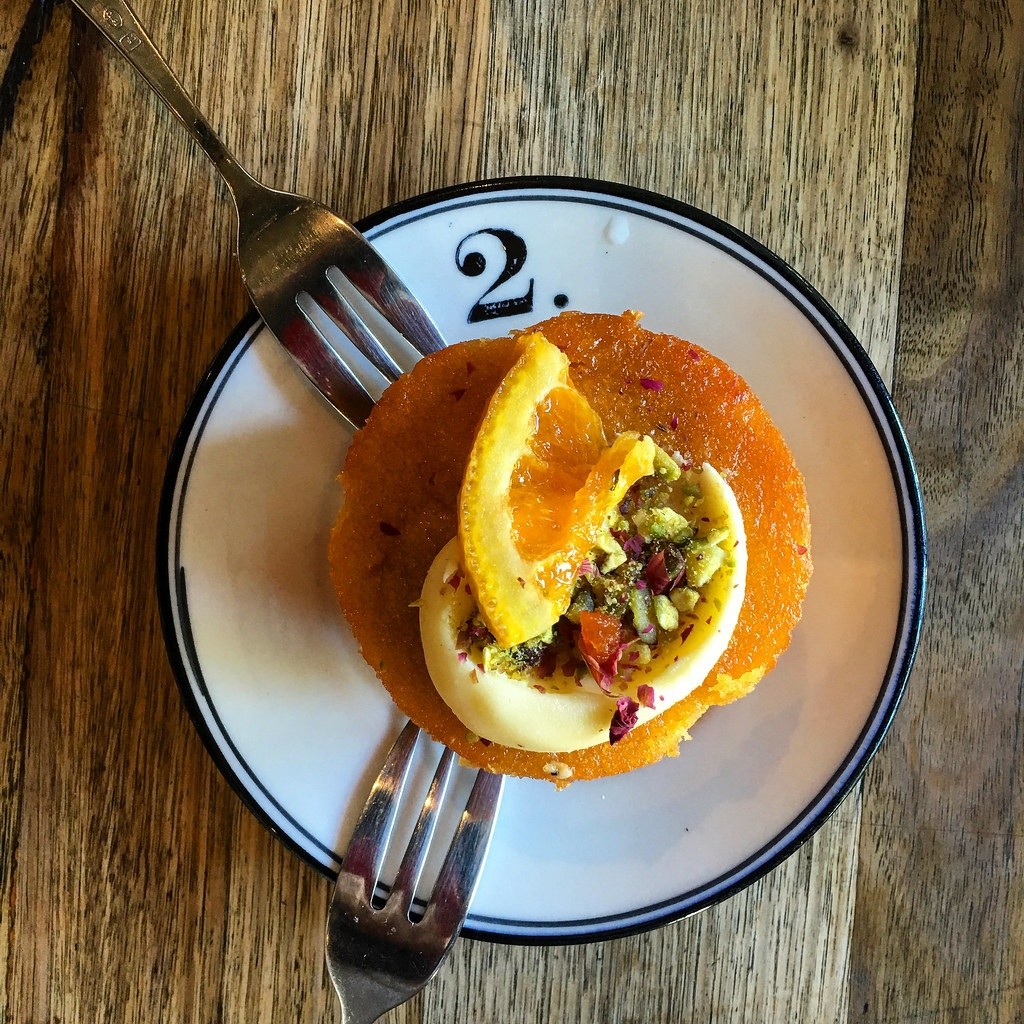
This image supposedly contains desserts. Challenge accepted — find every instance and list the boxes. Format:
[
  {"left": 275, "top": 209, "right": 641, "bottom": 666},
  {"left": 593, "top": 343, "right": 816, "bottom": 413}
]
[{"left": 329, "top": 312, "right": 815, "bottom": 780}]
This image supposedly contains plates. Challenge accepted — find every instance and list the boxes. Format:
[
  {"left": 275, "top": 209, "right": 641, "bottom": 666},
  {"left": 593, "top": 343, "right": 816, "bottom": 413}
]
[{"left": 152, "top": 177, "right": 928, "bottom": 946}]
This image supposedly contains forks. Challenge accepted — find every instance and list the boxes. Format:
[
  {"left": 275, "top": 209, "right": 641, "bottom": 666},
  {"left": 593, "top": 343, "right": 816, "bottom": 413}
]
[
  {"left": 325, "top": 718, "right": 504, "bottom": 1022},
  {"left": 74, "top": 1, "right": 447, "bottom": 432}
]
